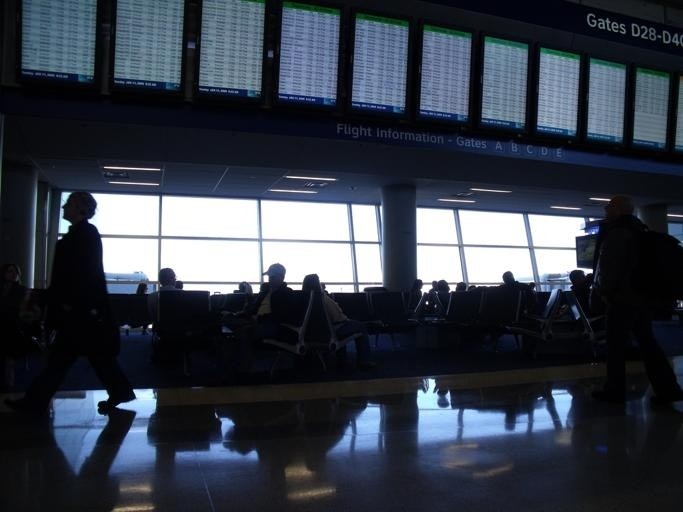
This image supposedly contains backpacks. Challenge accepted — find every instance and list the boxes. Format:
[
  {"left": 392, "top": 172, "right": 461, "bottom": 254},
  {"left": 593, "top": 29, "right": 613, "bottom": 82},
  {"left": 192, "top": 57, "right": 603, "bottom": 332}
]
[{"left": 638, "top": 222, "right": 682, "bottom": 320}]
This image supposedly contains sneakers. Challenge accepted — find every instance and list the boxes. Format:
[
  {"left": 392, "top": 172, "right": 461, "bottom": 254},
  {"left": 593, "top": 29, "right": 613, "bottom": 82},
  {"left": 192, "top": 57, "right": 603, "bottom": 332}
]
[
  {"left": 590, "top": 386, "right": 627, "bottom": 405},
  {"left": 650, "top": 388, "right": 683, "bottom": 407}
]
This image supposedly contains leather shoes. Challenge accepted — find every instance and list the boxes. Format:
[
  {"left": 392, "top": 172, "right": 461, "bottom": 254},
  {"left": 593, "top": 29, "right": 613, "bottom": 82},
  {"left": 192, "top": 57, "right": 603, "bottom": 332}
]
[
  {"left": 4, "top": 395, "right": 51, "bottom": 420},
  {"left": 98, "top": 392, "right": 136, "bottom": 408}
]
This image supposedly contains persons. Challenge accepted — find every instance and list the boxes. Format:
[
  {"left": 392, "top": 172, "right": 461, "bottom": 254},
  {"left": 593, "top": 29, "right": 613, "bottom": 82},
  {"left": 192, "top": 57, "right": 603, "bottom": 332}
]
[
  {"left": 570, "top": 271, "right": 594, "bottom": 295},
  {"left": 590, "top": 193, "right": 683, "bottom": 403},
  {"left": 144, "top": 263, "right": 377, "bottom": 393},
  {"left": 0, "top": 262, "right": 41, "bottom": 375},
  {"left": 410, "top": 279, "right": 518, "bottom": 312},
  {"left": 4, "top": 191, "right": 136, "bottom": 415}
]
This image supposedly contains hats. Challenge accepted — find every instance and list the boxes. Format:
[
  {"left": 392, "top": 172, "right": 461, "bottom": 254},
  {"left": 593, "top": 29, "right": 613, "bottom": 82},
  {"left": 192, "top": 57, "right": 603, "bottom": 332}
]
[
  {"left": 604, "top": 194, "right": 634, "bottom": 218},
  {"left": 262, "top": 264, "right": 286, "bottom": 279}
]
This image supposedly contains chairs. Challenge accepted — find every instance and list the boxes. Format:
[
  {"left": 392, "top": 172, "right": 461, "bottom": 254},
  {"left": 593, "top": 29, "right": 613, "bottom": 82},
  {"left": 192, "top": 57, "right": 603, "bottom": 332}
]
[
  {"left": 108, "top": 292, "right": 154, "bottom": 330},
  {"left": 314, "top": 287, "right": 451, "bottom": 372},
  {"left": 150, "top": 287, "right": 313, "bottom": 385},
  {"left": 420, "top": 289, "right": 608, "bottom": 366},
  {"left": 1, "top": 284, "right": 51, "bottom": 364}
]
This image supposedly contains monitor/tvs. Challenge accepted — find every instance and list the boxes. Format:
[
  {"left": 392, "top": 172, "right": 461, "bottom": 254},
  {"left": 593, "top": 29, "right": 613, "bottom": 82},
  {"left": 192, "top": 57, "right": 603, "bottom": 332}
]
[
  {"left": 575, "top": 233, "right": 599, "bottom": 267},
  {"left": 585, "top": 220, "right": 602, "bottom": 235}
]
